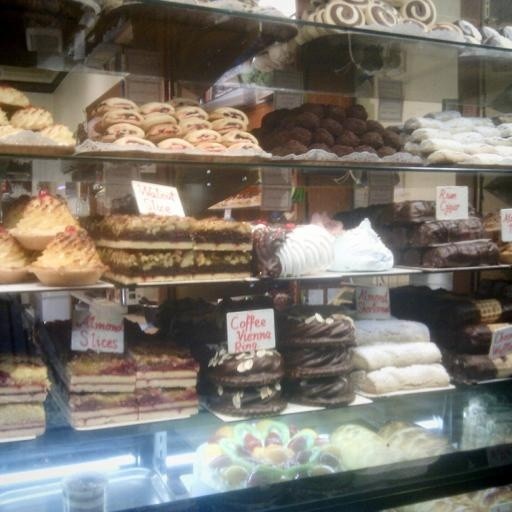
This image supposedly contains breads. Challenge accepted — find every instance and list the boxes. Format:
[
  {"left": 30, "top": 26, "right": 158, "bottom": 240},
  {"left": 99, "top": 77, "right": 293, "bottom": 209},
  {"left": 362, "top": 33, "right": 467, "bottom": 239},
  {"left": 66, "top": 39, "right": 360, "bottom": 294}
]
[
  {"left": 396, "top": 109, "right": 512, "bottom": 167},
  {"left": 248, "top": 103, "right": 403, "bottom": 156},
  {"left": 0, "top": 84, "right": 77, "bottom": 156},
  {"left": 86, "top": 97, "right": 265, "bottom": 155},
  {"left": 295, "top": 0, "right": 512, "bottom": 49},
  {"left": 333, "top": 418, "right": 512, "bottom": 512}
]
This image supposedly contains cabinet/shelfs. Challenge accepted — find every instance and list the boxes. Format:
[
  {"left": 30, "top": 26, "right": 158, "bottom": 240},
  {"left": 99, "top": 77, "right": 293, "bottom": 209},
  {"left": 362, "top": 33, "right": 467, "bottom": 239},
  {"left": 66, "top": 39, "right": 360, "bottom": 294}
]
[{"left": 1, "top": 1, "right": 510, "bottom": 512}]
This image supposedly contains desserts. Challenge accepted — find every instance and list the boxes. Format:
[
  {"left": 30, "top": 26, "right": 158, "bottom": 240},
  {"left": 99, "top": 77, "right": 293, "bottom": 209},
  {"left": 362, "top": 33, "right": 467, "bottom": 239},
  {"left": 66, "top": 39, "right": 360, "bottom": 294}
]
[
  {"left": 1, "top": 285, "right": 511, "bottom": 447},
  {"left": 0, "top": 187, "right": 512, "bottom": 287}
]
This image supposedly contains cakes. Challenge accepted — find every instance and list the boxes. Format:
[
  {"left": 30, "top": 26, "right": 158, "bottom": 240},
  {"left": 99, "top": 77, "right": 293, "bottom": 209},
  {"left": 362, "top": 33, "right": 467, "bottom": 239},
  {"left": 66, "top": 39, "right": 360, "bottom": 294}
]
[
  {"left": 62, "top": 474, "right": 106, "bottom": 512},
  {"left": 192, "top": 419, "right": 350, "bottom": 512}
]
